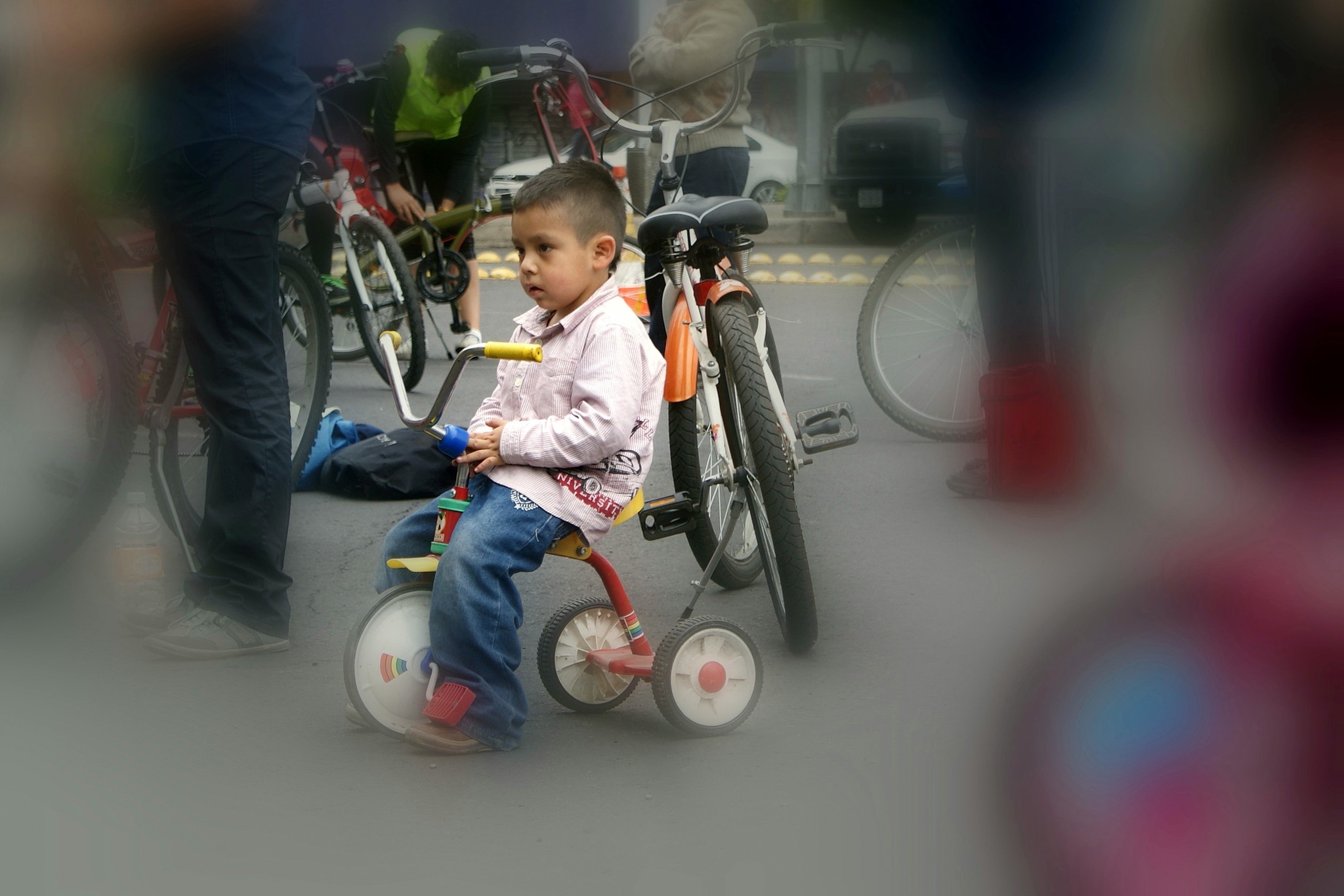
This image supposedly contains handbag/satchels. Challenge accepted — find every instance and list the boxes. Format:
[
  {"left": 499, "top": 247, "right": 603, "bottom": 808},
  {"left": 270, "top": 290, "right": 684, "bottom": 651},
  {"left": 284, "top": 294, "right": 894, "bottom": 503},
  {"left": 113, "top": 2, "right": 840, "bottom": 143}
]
[{"left": 321, "top": 422, "right": 467, "bottom": 499}]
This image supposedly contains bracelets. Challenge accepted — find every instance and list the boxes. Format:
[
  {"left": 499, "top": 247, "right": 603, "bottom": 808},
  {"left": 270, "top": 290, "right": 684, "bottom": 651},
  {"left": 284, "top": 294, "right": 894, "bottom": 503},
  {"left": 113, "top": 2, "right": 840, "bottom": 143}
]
[{"left": 437, "top": 207, "right": 450, "bottom": 214}]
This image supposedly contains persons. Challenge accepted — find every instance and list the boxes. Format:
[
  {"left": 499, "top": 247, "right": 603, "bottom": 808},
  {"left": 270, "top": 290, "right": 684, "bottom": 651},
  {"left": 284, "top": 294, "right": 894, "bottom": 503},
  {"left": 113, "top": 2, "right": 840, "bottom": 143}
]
[
  {"left": 372, "top": 27, "right": 493, "bottom": 359},
  {"left": 0, "top": 1, "right": 319, "bottom": 658},
  {"left": 344, "top": 156, "right": 666, "bottom": 755},
  {"left": 628, "top": 0, "right": 760, "bottom": 360}
]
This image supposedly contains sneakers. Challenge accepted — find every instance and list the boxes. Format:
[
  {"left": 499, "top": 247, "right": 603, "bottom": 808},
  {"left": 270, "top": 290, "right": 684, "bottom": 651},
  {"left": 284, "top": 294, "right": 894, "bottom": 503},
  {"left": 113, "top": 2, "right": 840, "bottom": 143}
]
[
  {"left": 945, "top": 458, "right": 988, "bottom": 498},
  {"left": 403, "top": 721, "right": 486, "bottom": 754},
  {"left": 456, "top": 328, "right": 483, "bottom": 360},
  {"left": 150, "top": 605, "right": 293, "bottom": 656},
  {"left": 395, "top": 336, "right": 430, "bottom": 361},
  {"left": 124, "top": 588, "right": 195, "bottom": 640}
]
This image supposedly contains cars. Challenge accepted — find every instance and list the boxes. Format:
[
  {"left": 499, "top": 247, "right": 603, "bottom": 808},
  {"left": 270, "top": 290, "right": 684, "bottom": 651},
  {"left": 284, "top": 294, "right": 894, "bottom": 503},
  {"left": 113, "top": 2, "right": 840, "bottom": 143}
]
[
  {"left": 824, "top": 76, "right": 978, "bottom": 229},
  {"left": 477, "top": 122, "right": 808, "bottom": 216}
]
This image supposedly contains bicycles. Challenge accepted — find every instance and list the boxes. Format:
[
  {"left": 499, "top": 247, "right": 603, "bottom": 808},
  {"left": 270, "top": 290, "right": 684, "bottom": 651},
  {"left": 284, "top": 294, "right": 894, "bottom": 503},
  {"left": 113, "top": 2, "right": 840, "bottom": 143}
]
[
  {"left": 851, "top": 205, "right": 997, "bottom": 450},
  {"left": 1, "top": 73, "right": 431, "bottom": 593},
  {"left": 458, "top": 20, "right": 892, "bottom": 647},
  {"left": 340, "top": 334, "right": 762, "bottom": 749}
]
